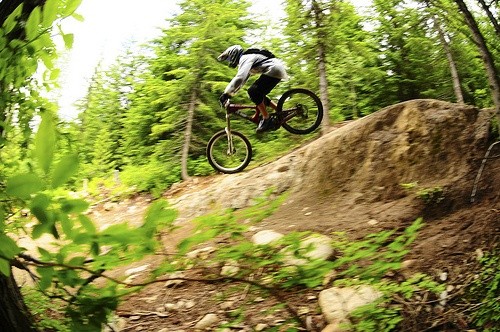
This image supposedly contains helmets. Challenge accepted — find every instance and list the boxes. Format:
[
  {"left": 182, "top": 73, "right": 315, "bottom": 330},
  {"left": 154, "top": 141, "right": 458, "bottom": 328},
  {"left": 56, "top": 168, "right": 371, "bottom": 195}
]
[{"left": 217, "top": 45, "right": 244, "bottom": 68}]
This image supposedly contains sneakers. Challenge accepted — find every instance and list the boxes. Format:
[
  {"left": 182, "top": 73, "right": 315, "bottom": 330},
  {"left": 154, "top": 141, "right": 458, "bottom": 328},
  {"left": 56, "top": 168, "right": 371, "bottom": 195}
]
[{"left": 256, "top": 120, "right": 274, "bottom": 131}]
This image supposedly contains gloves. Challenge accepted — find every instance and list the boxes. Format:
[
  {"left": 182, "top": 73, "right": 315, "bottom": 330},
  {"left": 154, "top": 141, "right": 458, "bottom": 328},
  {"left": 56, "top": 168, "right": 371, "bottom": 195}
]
[{"left": 220, "top": 94, "right": 231, "bottom": 106}]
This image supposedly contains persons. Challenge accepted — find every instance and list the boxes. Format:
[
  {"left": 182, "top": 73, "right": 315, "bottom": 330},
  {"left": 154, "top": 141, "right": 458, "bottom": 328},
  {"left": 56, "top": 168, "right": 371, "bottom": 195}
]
[{"left": 217, "top": 44, "right": 287, "bottom": 133}]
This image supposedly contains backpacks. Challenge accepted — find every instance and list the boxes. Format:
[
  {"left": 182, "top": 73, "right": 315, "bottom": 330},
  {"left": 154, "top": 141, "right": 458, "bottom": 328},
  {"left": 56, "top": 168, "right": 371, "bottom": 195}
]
[{"left": 241, "top": 47, "right": 275, "bottom": 67}]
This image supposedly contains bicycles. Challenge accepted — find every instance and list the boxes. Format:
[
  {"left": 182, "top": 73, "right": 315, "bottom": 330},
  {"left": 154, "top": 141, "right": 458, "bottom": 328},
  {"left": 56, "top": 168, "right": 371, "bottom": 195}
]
[{"left": 206, "top": 88, "right": 324, "bottom": 174}]
